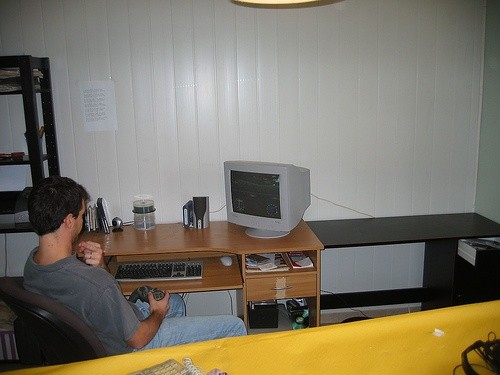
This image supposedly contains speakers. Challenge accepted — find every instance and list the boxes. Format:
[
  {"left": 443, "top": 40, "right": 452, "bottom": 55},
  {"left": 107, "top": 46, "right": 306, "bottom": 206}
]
[{"left": 192, "top": 196, "right": 210, "bottom": 229}]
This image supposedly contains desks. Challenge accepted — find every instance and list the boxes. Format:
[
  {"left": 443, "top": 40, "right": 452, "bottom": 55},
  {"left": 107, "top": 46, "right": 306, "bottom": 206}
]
[
  {"left": 304, "top": 212, "right": 500, "bottom": 311},
  {"left": 0, "top": 300, "right": 500, "bottom": 375},
  {"left": 73, "top": 218, "right": 325, "bottom": 335}
]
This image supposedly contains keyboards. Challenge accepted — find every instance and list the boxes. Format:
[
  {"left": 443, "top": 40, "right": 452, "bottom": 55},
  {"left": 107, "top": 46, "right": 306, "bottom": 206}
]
[{"left": 113, "top": 258, "right": 204, "bottom": 281}]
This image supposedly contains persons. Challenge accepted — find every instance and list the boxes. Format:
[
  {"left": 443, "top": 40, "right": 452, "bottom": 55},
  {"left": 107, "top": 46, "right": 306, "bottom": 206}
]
[{"left": 21, "top": 177, "right": 248, "bottom": 355}]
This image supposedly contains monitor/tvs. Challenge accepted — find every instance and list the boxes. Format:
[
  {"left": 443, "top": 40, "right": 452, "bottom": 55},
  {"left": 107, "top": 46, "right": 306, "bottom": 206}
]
[{"left": 223, "top": 160, "right": 310, "bottom": 238}]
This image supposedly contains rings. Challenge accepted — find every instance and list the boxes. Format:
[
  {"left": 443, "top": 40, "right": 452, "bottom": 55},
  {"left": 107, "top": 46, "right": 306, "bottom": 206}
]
[{"left": 89, "top": 251, "right": 94, "bottom": 259}]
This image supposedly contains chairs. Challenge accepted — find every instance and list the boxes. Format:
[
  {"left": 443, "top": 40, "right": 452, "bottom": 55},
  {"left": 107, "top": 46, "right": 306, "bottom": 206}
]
[{"left": 0, "top": 279, "right": 108, "bottom": 368}]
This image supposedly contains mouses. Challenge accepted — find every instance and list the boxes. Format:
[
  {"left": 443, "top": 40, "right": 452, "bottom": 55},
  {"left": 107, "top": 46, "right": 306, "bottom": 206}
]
[{"left": 219, "top": 255, "right": 233, "bottom": 266}]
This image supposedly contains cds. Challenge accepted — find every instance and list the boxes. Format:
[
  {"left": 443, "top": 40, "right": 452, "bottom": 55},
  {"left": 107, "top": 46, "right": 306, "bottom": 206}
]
[{"left": 133, "top": 199, "right": 154, "bottom": 227}]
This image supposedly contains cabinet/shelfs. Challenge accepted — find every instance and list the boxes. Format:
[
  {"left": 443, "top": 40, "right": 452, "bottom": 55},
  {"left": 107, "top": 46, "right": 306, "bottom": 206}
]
[{"left": 0, "top": 55, "right": 60, "bottom": 278}]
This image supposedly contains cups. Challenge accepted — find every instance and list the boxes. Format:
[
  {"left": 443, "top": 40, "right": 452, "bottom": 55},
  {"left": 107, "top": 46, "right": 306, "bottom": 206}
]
[{"left": 131, "top": 200, "right": 157, "bottom": 230}]
